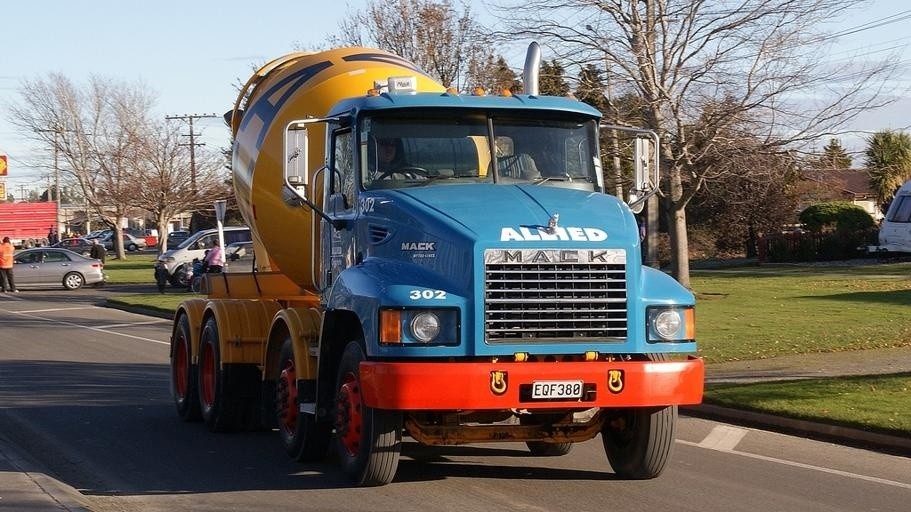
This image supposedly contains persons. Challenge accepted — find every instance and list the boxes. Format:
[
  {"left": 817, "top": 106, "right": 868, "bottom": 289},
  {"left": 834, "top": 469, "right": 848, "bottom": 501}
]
[
  {"left": 17, "top": 253, "right": 36, "bottom": 263},
  {"left": 186, "top": 257, "right": 204, "bottom": 293},
  {"left": 1, "top": 236, "right": 20, "bottom": 293},
  {"left": 201, "top": 250, "right": 210, "bottom": 273},
  {"left": 204, "top": 237, "right": 224, "bottom": 273},
  {"left": 91, "top": 238, "right": 106, "bottom": 286},
  {"left": 46, "top": 222, "right": 86, "bottom": 248},
  {"left": 343, "top": 131, "right": 436, "bottom": 208}
]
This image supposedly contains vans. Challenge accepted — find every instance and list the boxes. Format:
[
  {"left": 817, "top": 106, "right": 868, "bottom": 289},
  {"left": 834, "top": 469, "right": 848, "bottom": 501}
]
[
  {"left": 875, "top": 178, "right": 911, "bottom": 254},
  {"left": 150, "top": 226, "right": 250, "bottom": 292}
]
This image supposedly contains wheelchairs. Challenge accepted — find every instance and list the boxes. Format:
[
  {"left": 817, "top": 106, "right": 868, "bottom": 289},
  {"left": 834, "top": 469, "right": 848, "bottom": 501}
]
[{"left": 189, "top": 259, "right": 207, "bottom": 293}]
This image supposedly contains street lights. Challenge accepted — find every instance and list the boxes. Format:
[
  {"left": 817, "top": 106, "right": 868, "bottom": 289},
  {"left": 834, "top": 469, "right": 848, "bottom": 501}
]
[{"left": 585, "top": 23, "right": 626, "bottom": 200}]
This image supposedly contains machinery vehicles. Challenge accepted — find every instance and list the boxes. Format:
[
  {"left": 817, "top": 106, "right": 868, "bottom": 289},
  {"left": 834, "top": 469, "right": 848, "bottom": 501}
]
[{"left": 157, "top": 31, "right": 714, "bottom": 494}]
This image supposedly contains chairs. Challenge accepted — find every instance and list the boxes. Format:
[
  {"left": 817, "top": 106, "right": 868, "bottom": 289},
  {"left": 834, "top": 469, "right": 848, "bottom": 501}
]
[{"left": 485, "top": 136, "right": 542, "bottom": 185}]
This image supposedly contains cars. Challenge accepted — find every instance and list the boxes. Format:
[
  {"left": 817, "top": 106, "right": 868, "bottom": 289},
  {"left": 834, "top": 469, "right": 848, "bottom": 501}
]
[
  {"left": 223, "top": 238, "right": 255, "bottom": 261},
  {"left": 0, "top": 226, "right": 190, "bottom": 294}
]
